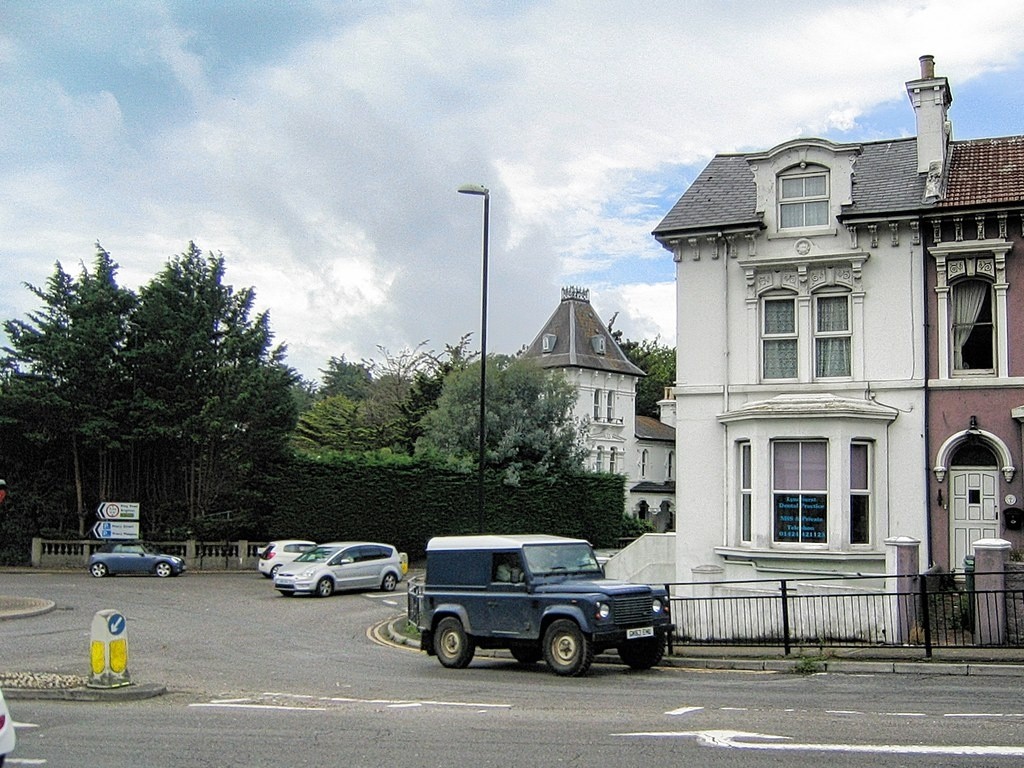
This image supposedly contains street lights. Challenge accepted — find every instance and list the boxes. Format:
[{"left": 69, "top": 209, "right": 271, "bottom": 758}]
[{"left": 457, "top": 184, "right": 490, "bottom": 540}]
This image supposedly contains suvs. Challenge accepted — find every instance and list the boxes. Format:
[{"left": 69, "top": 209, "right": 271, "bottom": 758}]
[{"left": 420, "top": 534, "right": 677, "bottom": 677}]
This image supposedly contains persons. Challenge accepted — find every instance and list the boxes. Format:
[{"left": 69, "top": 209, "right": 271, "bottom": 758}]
[{"left": 496, "top": 554, "right": 524, "bottom": 582}]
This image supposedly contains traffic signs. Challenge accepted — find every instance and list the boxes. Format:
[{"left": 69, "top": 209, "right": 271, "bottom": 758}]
[
  {"left": 93, "top": 521, "right": 139, "bottom": 539},
  {"left": 98, "top": 502, "right": 139, "bottom": 520}
]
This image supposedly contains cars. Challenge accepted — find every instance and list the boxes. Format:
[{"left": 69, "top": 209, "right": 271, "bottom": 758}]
[
  {"left": 272, "top": 542, "right": 407, "bottom": 597},
  {"left": 85, "top": 542, "right": 187, "bottom": 579},
  {"left": 0, "top": 687, "right": 17, "bottom": 766},
  {"left": 256, "top": 540, "right": 319, "bottom": 580}
]
